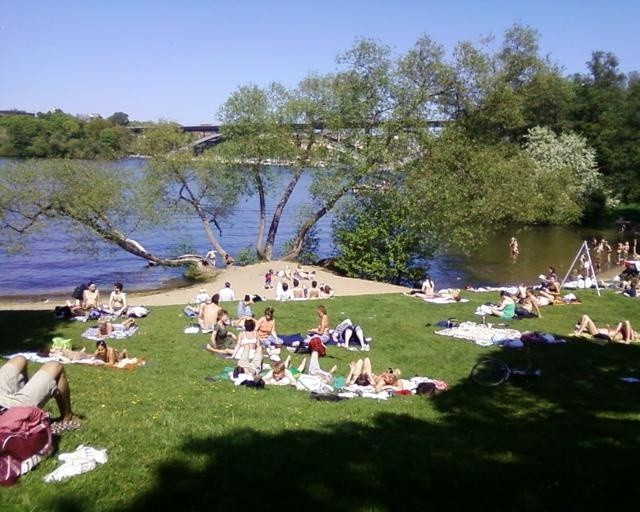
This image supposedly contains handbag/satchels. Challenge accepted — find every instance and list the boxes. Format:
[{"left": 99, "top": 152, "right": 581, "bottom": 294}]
[{"left": 1, "top": 406, "right": 56, "bottom": 486}]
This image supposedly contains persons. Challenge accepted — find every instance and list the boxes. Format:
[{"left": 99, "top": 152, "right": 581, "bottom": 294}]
[
  {"left": 490, "top": 236, "right": 640, "bottom": 345},
  {"left": 185, "top": 263, "right": 412, "bottom": 398},
  {"left": 204, "top": 249, "right": 219, "bottom": 266},
  {"left": 435, "top": 291, "right": 464, "bottom": 301},
  {"left": 0, "top": 356, "right": 85, "bottom": 429},
  {"left": 400, "top": 273, "right": 437, "bottom": 299},
  {"left": 35, "top": 279, "right": 136, "bottom": 367}
]
